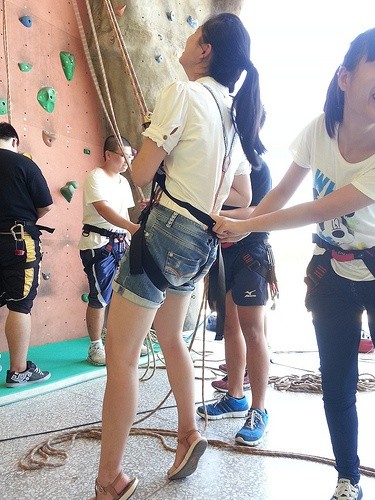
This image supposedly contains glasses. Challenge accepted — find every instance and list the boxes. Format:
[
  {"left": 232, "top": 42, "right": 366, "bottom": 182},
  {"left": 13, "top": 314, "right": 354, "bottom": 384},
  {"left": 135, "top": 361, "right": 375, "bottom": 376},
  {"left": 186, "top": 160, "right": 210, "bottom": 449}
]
[{"left": 112, "top": 149, "right": 134, "bottom": 162}]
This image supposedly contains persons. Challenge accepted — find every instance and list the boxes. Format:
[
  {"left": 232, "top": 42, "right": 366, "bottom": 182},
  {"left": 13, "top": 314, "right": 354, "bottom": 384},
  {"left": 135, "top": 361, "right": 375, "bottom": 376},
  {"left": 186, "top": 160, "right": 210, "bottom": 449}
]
[
  {"left": 92, "top": 12, "right": 261, "bottom": 500},
  {"left": 213, "top": 27, "right": 375, "bottom": 500},
  {"left": 197, "top": 105, "right": 273, "bottom": 445},
  {"left": 79, "top": 135, "right": 152, "bottom": 366},
  {"left": 0, "top": 122, "right": 53, "bottom": 388}
]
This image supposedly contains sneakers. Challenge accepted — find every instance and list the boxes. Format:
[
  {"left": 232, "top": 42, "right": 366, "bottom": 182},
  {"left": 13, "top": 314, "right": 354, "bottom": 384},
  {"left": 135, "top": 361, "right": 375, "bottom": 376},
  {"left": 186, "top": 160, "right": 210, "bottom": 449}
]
[
  {"left": 6, "top": 360, "right": 50, "bottom": 388},
  {"left": 141, "top": 344, "right": 150, "bottom": 355},
  {"left": 219, "top": 364, "right": 227, "bottom": 373},
  {"left": 196, "top": 392, "right": 251, "bottom": 421},
  {"left": 211, "top": 370, "right": 252, "bottom": 391},
  {"left": 88, "top": 349, "right": 106, "bottom": 366},
  {"left": 234, "top": 407, "right": 270, "bottom": 447},
  {"left": 329, "top": 478, "right": 363, "bottom": 500}
]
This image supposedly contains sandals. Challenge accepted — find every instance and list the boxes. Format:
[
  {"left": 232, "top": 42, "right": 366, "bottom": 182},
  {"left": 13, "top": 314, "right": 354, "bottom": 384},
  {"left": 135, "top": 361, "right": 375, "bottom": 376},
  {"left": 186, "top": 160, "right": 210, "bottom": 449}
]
[
  {"left": 95, "top": 469, "right": 140, "bottom": 500},
  {"left": 167, "top": 429, "right": 208, "bottom": 479}
]
